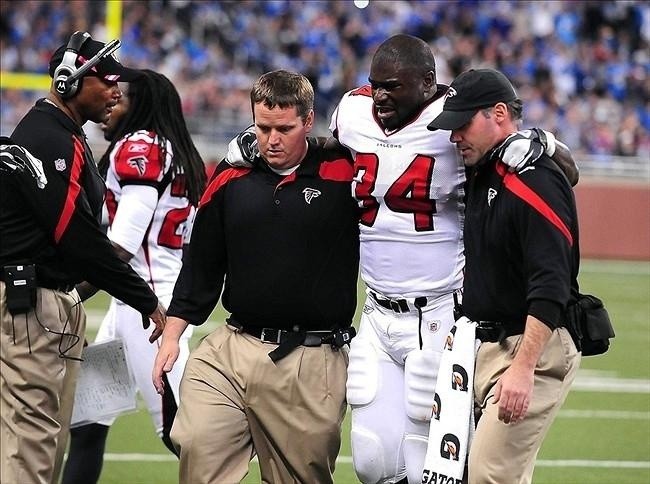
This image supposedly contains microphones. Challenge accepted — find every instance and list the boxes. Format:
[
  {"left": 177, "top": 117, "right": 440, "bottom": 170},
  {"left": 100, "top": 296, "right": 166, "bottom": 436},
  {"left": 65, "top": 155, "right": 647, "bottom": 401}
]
[{"left": 97, "top": 36, "right": 121, "bottom": 60}]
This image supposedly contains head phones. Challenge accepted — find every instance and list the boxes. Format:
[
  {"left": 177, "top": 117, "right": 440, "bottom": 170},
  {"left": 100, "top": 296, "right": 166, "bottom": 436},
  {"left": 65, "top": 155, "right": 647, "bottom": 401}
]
[{"left": 53, "top": 29, "right": 91, "bottom": 99}]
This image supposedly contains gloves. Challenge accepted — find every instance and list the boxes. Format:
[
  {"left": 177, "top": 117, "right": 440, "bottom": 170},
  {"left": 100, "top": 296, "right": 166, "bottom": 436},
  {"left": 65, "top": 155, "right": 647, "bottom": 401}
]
[
  {"left": 0, "top": 142, "right": 47, "bottom": 190},
  {"left": 224, "top": 121, "right": 260, "bottom": 168},
  {"left": 489, "top": 123, "right": 557, "bottom": 172}
]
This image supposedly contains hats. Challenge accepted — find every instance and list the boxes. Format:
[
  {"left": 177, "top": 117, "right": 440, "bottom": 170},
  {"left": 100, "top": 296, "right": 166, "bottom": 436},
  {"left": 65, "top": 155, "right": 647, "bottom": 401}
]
[
  {"left": 48, "top": 40, "right": 139, "bottom": 82},
  {"left": 427, "top": 67, "right": 519, "bottom": 132}
]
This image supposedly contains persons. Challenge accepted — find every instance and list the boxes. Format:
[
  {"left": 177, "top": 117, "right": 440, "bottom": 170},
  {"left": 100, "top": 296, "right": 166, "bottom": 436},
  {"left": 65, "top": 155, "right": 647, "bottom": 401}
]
[
  {"left": 0, "top": 39, "right": 165, "bottom": 484},
  {"left": 427, "top": 65, "right": 578, "bottom": 484},
  {"left": 62, "top": 69, "right": 206, "bottom": 484},
  {"left": 328, "top": 36, "right": 580, "bottom": 484},
  {"left": 151, "top": 71, "right": 358, "bottom": 483}
]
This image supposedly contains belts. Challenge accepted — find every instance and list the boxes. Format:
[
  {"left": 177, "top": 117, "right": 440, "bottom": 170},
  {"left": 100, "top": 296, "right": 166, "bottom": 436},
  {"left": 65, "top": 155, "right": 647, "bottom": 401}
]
[
  {"left": 228, "top": 314, "right": 333, "bottom": 362},
  {"left": 370, "top": 290, "right": 428, "bottom": 349},
  {"left": 39, "top": 275, "right": 79, "bottom": 291},
  {"left": 475, "top": 320, "right": 530, "bottom": 342}
]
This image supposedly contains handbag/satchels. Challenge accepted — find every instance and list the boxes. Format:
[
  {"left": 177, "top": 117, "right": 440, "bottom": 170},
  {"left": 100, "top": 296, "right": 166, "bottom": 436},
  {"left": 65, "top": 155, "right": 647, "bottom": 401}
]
[{"left": 568, "top": 292, "right": 615, "bottom": 356}]
